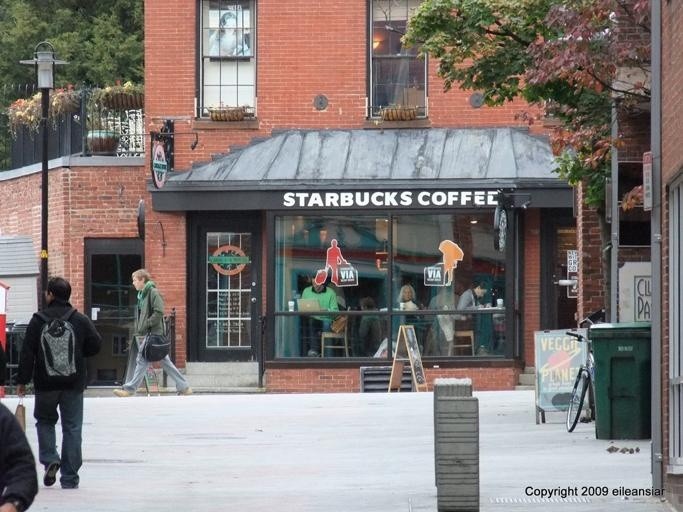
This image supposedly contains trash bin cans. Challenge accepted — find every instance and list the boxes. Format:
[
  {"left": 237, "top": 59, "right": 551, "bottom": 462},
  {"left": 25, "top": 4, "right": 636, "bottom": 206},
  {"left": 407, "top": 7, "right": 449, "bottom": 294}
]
[{"left": 589, "top": 321, "right": 652, "bottom": 439}]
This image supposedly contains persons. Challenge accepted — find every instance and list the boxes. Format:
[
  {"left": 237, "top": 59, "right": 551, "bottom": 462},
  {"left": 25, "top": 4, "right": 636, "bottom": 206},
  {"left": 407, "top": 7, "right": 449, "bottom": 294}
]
[
  {"left": 16, "top": 277, "right": 102, "bottom": 490},
  {"left": 0, "top": 400, "right": 40, "bottom": 512},
  {"left": 112, "top": 270, "right": 194, "bottom": 396},
  {"left": 298, "top": 268, "right": 341, "bottom": 357},
  {"left": 357, "top": 297, "right": 385, "bottom": 357},
  {"left": 451, "top": 281, "right": 492, "bottom": 357},
  {"left": 398, "top": 284, "right": 425, "bottom": 312}
]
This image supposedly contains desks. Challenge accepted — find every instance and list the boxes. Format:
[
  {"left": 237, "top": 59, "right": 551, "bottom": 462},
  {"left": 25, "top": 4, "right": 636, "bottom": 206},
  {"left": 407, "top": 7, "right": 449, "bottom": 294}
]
[{"left": 274, "top": 307, "right": 508, "bottom": 356}]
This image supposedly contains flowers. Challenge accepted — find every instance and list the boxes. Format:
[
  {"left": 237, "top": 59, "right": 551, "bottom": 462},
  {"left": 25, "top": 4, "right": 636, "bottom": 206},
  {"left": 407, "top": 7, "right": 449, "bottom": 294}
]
[
  {"left": 9, "top": 82, "right": 144, "bottom": 109},
  {"left": 1, "top": 108, "right": 61, "bottom": 142}
]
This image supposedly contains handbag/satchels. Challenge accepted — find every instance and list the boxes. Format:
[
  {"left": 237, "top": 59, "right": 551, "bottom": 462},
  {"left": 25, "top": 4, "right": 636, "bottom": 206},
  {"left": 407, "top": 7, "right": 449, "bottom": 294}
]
[
  {"left": 142, "top": 289, "right": 170, "bottom": 362},
  {"left": 14, "top": 394, "right": 26, "bottom": 433}
]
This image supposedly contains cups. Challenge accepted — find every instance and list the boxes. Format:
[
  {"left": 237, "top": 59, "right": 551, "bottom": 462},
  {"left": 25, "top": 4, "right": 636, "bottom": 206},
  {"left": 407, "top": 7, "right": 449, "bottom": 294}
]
[
  {"left": 289, "top": 302, "right": 294, "bottom": 312},
  {"left": 400, "top": 303, "right": 405, "bottom": 311},
  {"left": 497, "top": 299, "right": 503, "bottom": 308}
]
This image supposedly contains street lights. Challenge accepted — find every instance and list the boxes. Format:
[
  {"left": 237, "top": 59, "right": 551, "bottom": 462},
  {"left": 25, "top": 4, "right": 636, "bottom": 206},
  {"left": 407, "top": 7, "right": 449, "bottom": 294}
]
[{"left": 18, "top": 41, "right": 69, "bottom": 309}]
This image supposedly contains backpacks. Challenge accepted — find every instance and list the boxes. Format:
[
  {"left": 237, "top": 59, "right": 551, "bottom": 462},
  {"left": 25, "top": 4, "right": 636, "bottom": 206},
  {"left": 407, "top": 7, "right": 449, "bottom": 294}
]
[{"left": 31, "top": 307, "right": 79, "bottom": 384}]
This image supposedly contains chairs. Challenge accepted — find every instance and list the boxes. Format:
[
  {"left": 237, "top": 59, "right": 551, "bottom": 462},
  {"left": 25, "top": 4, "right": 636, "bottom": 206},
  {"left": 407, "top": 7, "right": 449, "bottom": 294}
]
[
  {"left": 446, "top": 306, "right": 475, "bottom": 358},
  {"left": 320, "top": 306, "right": 351, "bottom": 357}
]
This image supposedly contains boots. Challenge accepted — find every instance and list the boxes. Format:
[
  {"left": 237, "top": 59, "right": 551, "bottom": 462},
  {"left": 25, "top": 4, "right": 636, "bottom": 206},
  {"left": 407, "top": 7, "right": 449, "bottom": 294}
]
[
  {"left": 114, "top": 386, "right": 133, "bottom": 397},
  {"left": 176, "top": 385, "right": 194, "bottom": 396}
]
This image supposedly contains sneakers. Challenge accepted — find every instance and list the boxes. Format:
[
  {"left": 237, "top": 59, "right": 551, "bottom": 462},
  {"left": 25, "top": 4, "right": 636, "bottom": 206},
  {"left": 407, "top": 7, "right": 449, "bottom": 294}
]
[
  {"left": 62, "top": 481, "right": 78, "bottom": 489},
  {"left": 43, "top": 461, "right": 59, "bottom": 486}
]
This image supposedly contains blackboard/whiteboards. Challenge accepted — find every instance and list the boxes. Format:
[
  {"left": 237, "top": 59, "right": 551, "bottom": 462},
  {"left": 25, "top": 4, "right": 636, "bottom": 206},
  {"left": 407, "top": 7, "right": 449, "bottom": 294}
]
[
  {"left": 402, "top": 325, "right": 428, "bottom": 387},
  {"left": 534, "top": 328, "right": 589, "bottom": 412}
]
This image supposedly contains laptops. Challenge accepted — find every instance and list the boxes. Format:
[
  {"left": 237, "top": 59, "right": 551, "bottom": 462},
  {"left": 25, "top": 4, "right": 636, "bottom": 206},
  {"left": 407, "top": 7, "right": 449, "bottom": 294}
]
[{"left": 297, "top": 298, "right": 328, "bottom": 311}]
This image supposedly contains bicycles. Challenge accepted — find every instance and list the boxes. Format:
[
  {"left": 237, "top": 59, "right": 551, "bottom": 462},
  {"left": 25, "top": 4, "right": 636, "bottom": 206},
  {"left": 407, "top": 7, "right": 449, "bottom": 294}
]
[{"left": 565, "top": 332, "right": 596, "bottom": 432}]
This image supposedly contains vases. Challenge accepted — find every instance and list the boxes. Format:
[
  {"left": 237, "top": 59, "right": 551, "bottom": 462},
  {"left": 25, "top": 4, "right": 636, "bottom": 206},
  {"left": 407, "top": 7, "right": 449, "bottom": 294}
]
[{"left": 86, "top": 130, "right": 120, "bottom": 157}]
